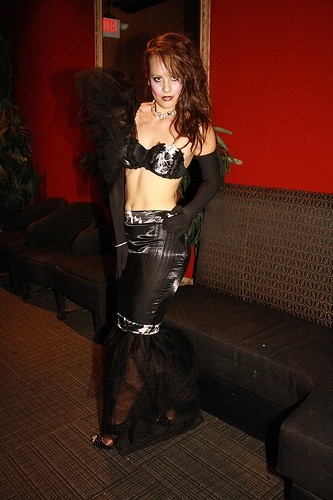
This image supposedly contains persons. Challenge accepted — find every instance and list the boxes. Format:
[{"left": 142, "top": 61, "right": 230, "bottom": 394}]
[{"left": 91, "top": 34, "right": 221, "bottom": 449}]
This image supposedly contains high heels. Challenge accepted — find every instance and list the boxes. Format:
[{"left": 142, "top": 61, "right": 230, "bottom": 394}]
[
  {"left": 155, "top": 403, "right": 181, "bottom": 427},
  {"left": 91, "top": 409, "right": 138, "bottom": 450}
]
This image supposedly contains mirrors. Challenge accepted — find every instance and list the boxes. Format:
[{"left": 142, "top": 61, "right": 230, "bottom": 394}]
[{"left": 93, "top": 0, "right": 211, "bottom": 149}]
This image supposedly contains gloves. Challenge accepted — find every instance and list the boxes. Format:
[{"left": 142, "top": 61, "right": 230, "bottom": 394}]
[
  {"left": 162, "top": 152, "right": 220, "bottom": 237},
  {"left": 109, "top": 169, "right": 128, "bottom": 278}
]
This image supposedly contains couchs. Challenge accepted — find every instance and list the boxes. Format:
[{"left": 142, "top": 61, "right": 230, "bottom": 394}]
[
  {"left": 0, "top": 198, "right": 67, "bottom": 295},
  {"left": 13, "top": 206, "right": 103, "bottom": 311},
  {"left": 55, "top": 222, "right": 116, "bottom": 343},
  {"left": 159, "top": 182, "right": 333, "bottom": 500}
]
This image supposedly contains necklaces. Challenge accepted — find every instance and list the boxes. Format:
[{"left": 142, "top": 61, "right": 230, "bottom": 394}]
[{"left": 151, "top": 99, "right": 176, "bottom": 119}]
